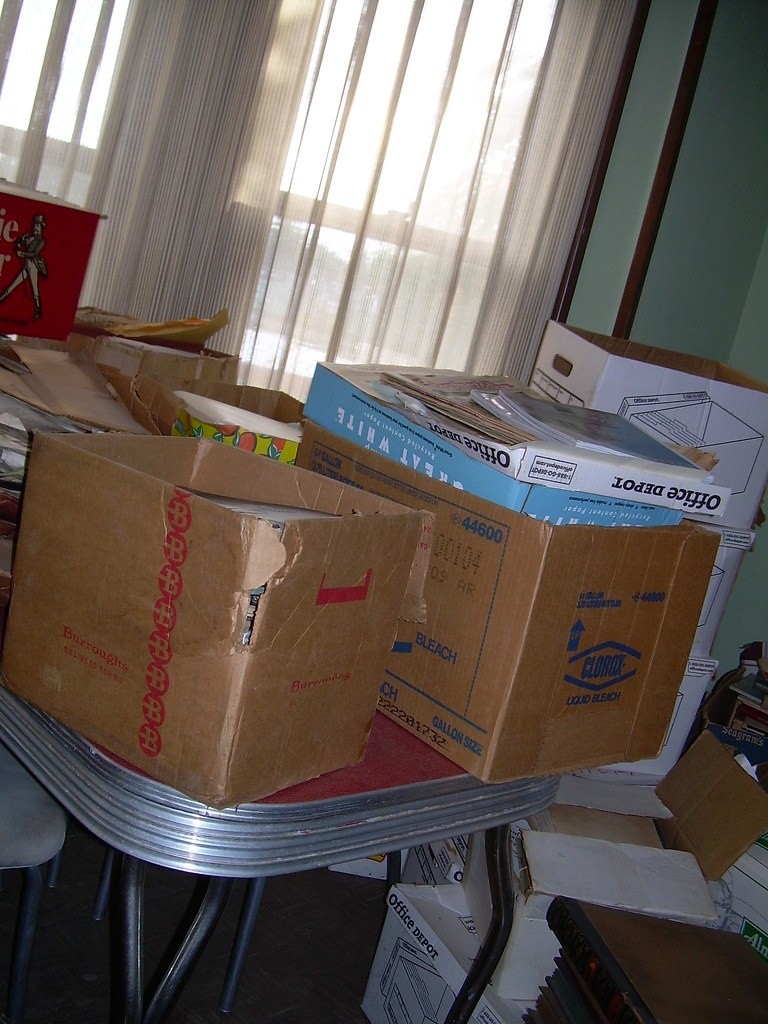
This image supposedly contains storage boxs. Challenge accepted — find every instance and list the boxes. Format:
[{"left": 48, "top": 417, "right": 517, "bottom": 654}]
[
  {"left": 525, "top": 318, "right": 767, "bottom": 531},
  {"left": 687, "top": 523, "right": 757, "bottom": 658},
  {"left": 0, "top": 428, "right": 433, "bottom": 808},
  {"left": 68, "top": 328, "right": 306, "bottom": 465},
  {"left": 302, "top": 363, "right": 685, "bottom": 528},
  {"left": 296, "top": 418, "right": 720, "bottom": 782},
  {"left": 330, "top": 654, "right": 768, "bottom": 1023},
  {"left": 0, "top": 183, "right": 108, "bottom": 340}
]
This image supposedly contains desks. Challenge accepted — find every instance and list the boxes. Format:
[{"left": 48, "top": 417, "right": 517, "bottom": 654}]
[{"left": 0, "top": 681, "right": 560, "bottom": 1024}]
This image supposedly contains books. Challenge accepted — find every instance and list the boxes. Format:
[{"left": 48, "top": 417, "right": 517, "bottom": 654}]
[{"left": 380, "top": 371, "right": 718, "bottom": 485}]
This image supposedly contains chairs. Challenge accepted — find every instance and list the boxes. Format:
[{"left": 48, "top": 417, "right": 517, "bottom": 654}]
[{"left": 0, "top": 742, "right": 67, "bottom": 1024}]
